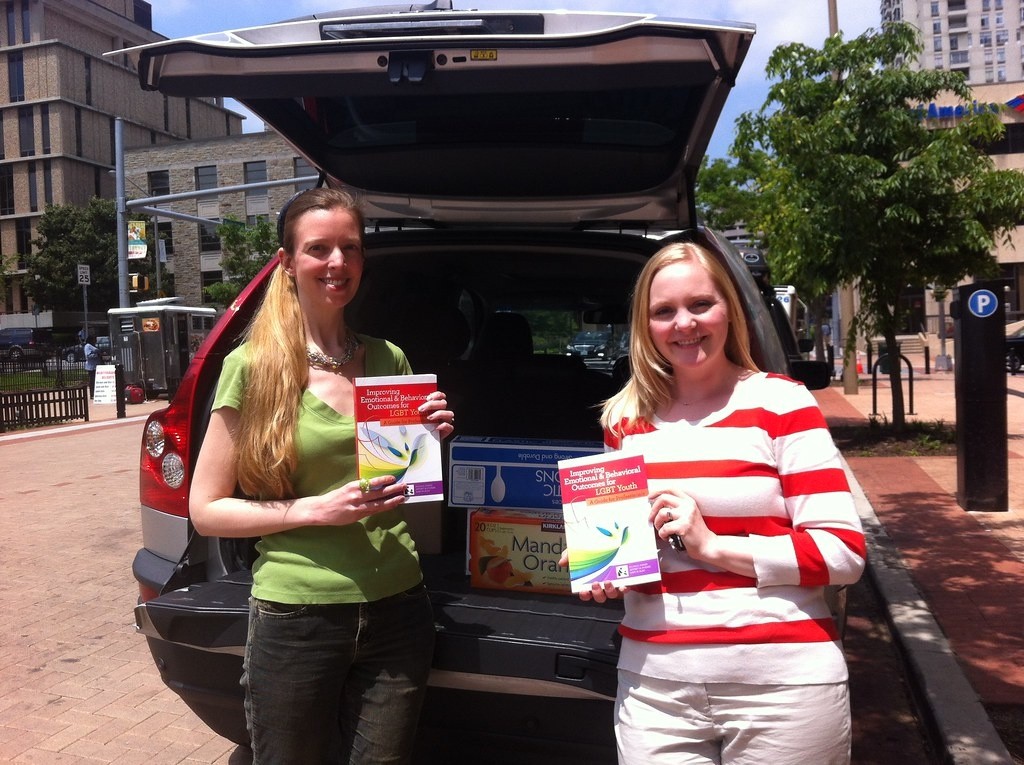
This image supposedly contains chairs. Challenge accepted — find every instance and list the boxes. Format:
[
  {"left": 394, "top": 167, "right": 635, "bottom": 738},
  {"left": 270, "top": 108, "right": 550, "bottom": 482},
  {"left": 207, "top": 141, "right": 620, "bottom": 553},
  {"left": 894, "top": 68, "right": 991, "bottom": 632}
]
[{"left": 468, "top": 313, "right": 541, "bottom": 382}]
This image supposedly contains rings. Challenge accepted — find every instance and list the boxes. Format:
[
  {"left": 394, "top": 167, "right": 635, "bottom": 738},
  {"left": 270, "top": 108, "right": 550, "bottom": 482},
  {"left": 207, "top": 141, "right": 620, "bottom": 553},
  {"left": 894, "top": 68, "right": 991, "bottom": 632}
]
[
  {"left": 665, "top": 508, "right": 674, "bottom": 522},
  {"left": 358, "top": 479, "right": 374, "bottom": 493}
]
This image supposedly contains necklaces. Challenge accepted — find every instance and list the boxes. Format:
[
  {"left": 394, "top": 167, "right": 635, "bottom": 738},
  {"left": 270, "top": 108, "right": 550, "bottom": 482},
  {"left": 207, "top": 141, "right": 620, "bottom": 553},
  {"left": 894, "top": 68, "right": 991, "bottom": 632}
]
[
  {"left": 673, "top": 397, "right": 700, "bottom": 408},
  {"left": 305, "top": 333, "right": 361, "bottom": 370}
]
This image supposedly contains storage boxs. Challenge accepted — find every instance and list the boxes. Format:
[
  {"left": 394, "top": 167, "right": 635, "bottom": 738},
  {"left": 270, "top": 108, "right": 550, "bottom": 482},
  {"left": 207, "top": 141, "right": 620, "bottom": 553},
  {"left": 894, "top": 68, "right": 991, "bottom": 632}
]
[{"left": 398, "top": 435, "right": 605, "bottom": 597}]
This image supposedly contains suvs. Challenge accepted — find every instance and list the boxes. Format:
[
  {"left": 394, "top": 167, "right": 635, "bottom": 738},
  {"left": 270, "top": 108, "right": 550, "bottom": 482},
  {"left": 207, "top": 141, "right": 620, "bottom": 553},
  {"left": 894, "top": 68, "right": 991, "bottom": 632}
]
[{"left": 0, "top": 327, "right": 56, "bottom": 363}]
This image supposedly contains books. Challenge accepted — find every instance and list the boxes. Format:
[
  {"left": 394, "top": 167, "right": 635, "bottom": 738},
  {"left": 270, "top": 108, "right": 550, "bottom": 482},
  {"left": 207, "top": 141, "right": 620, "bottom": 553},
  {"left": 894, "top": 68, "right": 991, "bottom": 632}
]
[
  {"left": 352, "top": 375, "right": 445, "bottom": 504},
  {"left": 557, "top": 446, "right": 662, "bottom": 593}
]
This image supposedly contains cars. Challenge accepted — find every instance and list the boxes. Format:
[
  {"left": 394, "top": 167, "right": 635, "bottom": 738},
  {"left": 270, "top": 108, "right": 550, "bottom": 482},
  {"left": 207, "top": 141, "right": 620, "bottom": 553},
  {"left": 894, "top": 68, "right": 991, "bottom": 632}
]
[
  {"left": 102, "top": 0, "right": 833, "bottom": 747},
  {"left": 1005, "top": 320, "right": 1024, "bottom": 373},
  {"left": 565, "top": 330, "right": 622, "bottom": 361},
  {"left": 59, "top": 336, "right": 113, "bottom": 363}
]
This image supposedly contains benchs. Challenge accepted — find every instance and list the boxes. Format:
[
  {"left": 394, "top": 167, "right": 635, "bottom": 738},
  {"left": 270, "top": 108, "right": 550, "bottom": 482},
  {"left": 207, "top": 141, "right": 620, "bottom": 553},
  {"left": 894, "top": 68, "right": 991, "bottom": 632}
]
[{"left": 356, "top": 291, "right": 478, "bottom": 399}]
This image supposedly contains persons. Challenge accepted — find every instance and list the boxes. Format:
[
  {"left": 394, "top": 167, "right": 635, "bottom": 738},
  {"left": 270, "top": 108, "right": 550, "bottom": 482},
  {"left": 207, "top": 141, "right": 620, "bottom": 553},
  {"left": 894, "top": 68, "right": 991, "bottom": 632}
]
[
  {"left": 559, "top": 243, "right": 866, "bottom": 765},
  {"left": 83, "top": 334, "right": 101, "bottom": 399},
  {"left": 189, "top": 189, "right": 452, "bottom": 765}
]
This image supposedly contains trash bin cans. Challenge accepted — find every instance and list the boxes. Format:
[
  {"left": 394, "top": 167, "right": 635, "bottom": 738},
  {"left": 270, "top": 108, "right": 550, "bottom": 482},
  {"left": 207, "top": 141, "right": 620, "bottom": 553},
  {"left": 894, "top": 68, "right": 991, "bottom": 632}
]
[{"left": 877, "top": 339, "right": 902, "bottom": 374}]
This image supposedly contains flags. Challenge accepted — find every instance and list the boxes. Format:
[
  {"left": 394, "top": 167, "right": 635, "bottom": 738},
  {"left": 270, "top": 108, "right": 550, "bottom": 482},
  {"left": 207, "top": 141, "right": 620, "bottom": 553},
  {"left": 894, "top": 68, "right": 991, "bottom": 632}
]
[{"left": 127, "top": 220, "right": 147, "bottom": 259}]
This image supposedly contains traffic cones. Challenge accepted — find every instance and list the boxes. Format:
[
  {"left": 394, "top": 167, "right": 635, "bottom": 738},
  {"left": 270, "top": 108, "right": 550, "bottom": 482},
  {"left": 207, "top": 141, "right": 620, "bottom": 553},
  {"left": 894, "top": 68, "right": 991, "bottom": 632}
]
[{"left": 856, "top": 351, "right": 864, "bottom": 373}]
